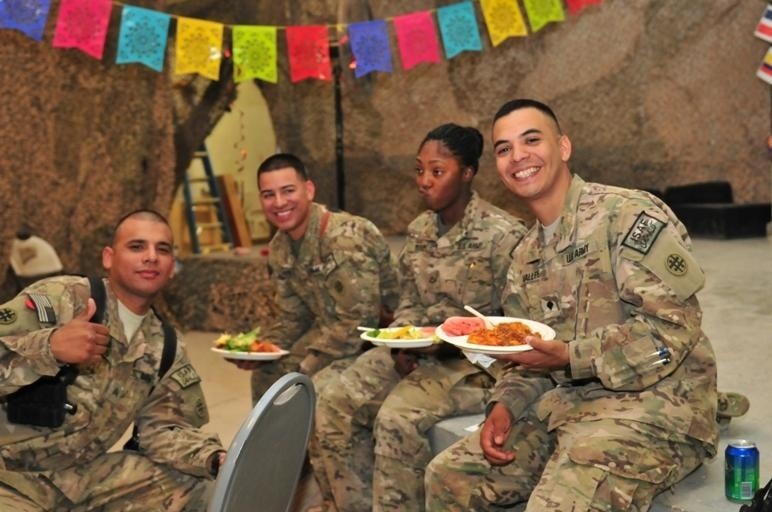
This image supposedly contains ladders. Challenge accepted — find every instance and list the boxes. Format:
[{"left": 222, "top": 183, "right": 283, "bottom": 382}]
[{"left": 172, "top": 103, "right": 233, "bottom": 255}]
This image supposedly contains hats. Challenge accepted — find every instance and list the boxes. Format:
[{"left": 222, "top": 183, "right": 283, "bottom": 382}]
[{"left": 717, "top": 391, "right": 750, "bottom": 428}]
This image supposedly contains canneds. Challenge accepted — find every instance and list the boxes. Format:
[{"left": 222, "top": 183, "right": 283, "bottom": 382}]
[{"left": 725, "top": 441, "right": 760, "bottom": 504}]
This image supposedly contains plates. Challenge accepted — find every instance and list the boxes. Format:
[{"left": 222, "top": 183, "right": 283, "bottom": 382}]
[
  {"left": 360, "top": 326, "right": 436, "bottom": 348},
  {"left": 436, "top": 315, "right": 557, "bottom": 355},
  {"left": 210, "top": 346, "right": 290, "bottom": 361}
]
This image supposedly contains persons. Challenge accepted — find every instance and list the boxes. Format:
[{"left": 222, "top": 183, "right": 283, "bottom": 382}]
[
  {"left": 421, "top": 98, "right": 722, "bottom": 512},
  {"left": 1, "top": 210, "right": 228, "bottom": 512},
  {"left": 221, "top": 152, "right": 399, "bottom": 511},
  {"left": 314, "top": 123, "right": 531, "bottom": 511}
]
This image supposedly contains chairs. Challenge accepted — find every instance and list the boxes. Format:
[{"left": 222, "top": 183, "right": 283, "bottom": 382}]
[{"left": 209, "top": 372, "right": 315, "bottom": 512}]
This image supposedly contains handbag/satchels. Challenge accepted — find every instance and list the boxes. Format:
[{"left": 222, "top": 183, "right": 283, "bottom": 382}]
[{"left": 7, "top": 375, "right": 67, "bottom": 427}]
